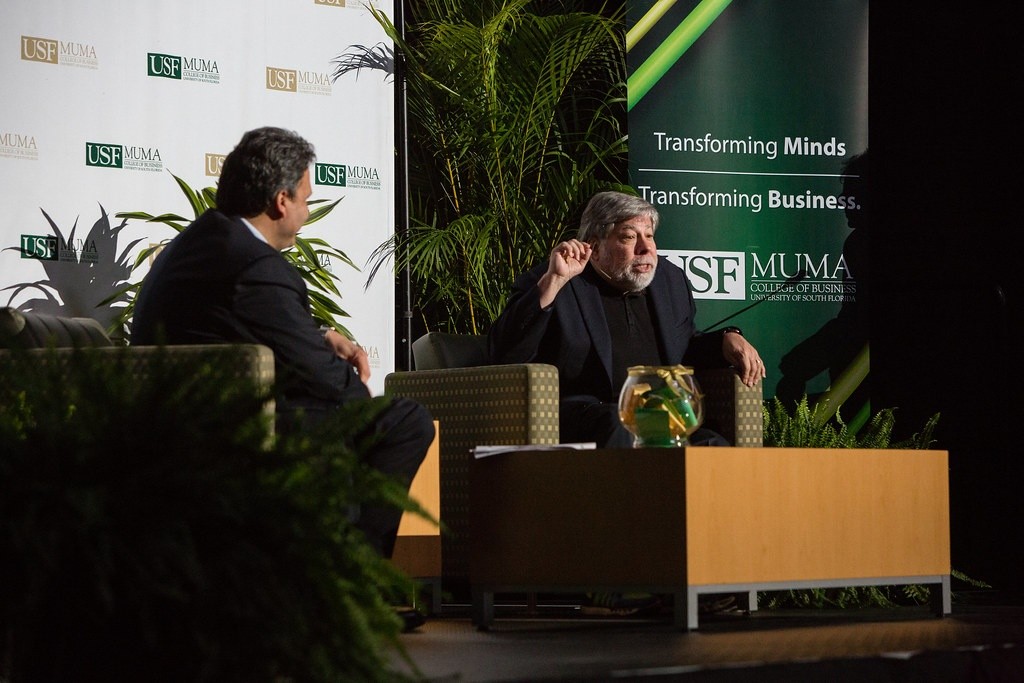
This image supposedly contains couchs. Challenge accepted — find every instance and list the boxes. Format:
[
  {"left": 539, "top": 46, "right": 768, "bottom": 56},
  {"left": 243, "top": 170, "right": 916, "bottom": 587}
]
[
  {"left": 384, "top": 332, "right": 762, "bottom": 611},
  {"left": 0, "top": 305, "right": 276, "bottom": 455}
]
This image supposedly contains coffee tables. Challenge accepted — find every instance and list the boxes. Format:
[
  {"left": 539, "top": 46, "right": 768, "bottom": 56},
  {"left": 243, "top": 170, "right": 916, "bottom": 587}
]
[{"left": 470, "top": 445, "right": 952, "bottom": 630}]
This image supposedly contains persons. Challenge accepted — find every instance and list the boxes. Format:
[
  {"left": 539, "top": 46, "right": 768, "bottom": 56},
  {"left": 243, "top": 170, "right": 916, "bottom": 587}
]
[
  {"left": 131, "top": 127, "right": 437, "bottom": 631},
  {"left": 486, "top": 192, "right": 767, "bottom": 446}
]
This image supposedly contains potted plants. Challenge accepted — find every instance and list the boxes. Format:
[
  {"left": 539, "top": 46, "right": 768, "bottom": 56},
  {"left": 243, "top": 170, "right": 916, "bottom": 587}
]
[{"left": 0, "top": 314, "right": 457, "bottom": 683}]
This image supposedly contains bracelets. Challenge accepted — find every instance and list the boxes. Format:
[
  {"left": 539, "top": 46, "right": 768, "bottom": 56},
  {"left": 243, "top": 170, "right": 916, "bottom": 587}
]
[{"left": 724, "top": 328, "right": 740, "bottom": 334}]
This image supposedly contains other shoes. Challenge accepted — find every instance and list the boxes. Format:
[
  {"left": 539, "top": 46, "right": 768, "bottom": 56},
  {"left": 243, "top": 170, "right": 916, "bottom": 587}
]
[
  {"left": 653, "top": 593, "right": 738, "bottom": 619},
  {"left": 385, "top": 602, "right": 427, "bottom": 633}
]
